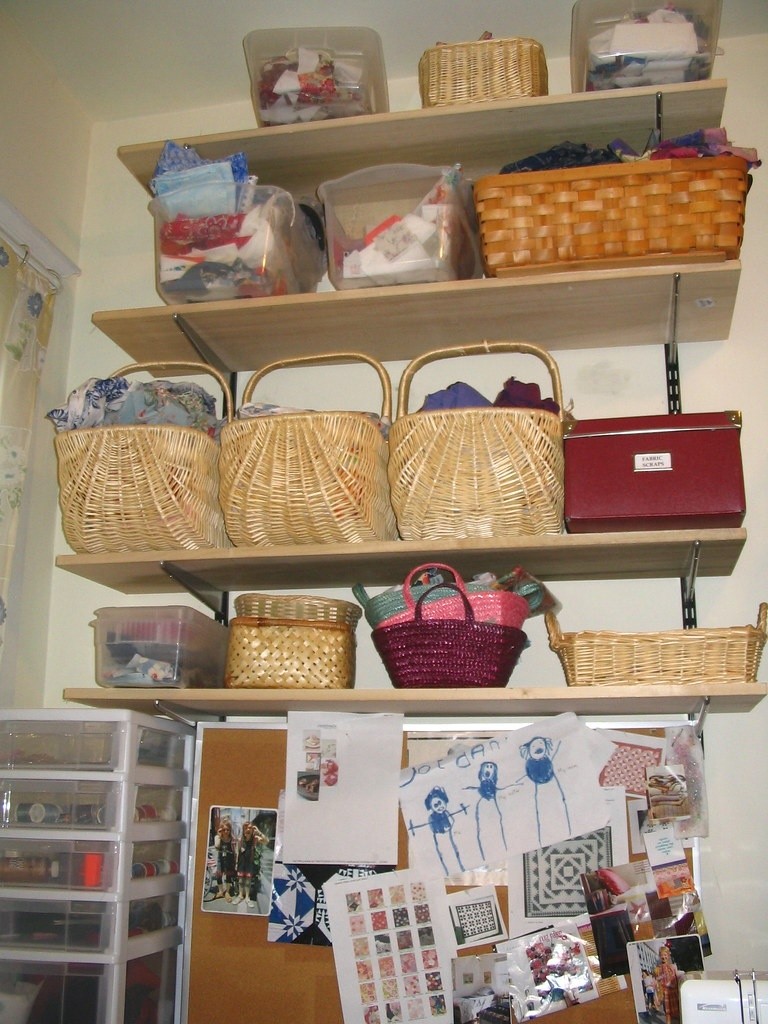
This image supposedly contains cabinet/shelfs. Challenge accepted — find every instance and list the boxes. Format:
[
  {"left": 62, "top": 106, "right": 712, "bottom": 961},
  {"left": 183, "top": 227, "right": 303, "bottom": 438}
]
[
  {"left": 52, "top": 77, "right": 766, "bottom": 716},
  {"left": 0, "top": 709, "right": 197, "bottom": 1024}
]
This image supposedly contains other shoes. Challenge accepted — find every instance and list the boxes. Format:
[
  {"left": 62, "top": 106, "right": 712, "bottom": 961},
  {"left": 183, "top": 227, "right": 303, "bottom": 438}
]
[
  {"left": 216, "top": 891, "right": 224, "bottom": 898},
  {"left": 232, "top": 896, "right": 243, "bottom": 905},
  {"left": 245, "top": 897, "right": 254, "bottom": 907},
  {"left": 225, "top": 892, "right": 232, "bottom": 903}
]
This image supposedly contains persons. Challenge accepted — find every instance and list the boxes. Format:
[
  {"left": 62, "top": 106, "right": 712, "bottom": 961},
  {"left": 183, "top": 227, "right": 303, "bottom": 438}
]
[
  {"left": 214, "top": 820, "right": 236, "bottom": 902},
  {"left": 637, "top": 971, "right": 661, "bottom": 1013},
  {"left": 656, "top": 947, "right": 682, "bottom": 1024},
  {"left": 233, "top": 822, "right": 269, "bottom": 907}
]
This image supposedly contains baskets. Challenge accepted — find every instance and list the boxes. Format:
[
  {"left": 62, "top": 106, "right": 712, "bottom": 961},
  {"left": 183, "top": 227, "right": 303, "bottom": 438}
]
[
  {"left": 54, "top": 362, "right": 234, "bottom": 554},
  {"left": 219, "top": 352, "right": 399, "bottom": 547},
  {"left": 352, "top": 564, "right": 544, "bottom": 688},
  {"left": 387, "top": 341, "right": 565, "bottom": 540},
  {"left": 224, "top": 593, "right": 362, "bottom": 688},
  {"left": 473, "top": 156, "right": 748, "bottom": 277},
  {"left": 545, "top": 602, "right": 768, "bottom": 685},
  {"left": 418, "top": 37, "right": 549, "bottom": 108}
]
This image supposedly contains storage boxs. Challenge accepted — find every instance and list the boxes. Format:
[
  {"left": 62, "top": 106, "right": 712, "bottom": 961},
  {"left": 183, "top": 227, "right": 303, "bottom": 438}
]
[
  {"left": 88, "top": 605, "right": 230, "bottom": 688},
  {"left": 317, "top": 162, "right": 482, "bottom": 290},
  {"left": 146, "top": 180, "right": 323, "bottom": 306},
  {"left": 562, "top": 409, "right": 747, "bottom": 532},
  {"left": 569, "top": 0, "right": 723, "bottom": 94},
  {"left": 242, "top": 27, "right": 390, "bottom": 128}
]
